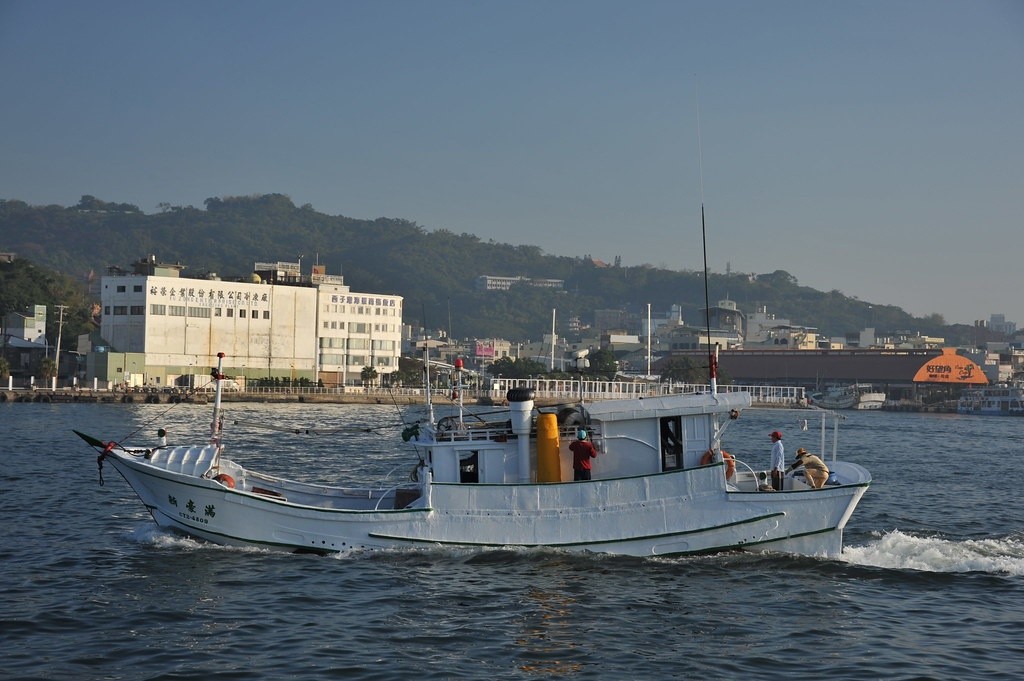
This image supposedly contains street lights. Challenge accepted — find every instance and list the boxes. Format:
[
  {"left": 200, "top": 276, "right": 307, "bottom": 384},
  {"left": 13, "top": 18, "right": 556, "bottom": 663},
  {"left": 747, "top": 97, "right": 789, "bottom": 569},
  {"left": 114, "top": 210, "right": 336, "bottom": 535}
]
[
  {"left": 290, "top": 365, "right": 294, "bottom": 391},
  {"left": 188, "top": 363, "right": 192, "bottom": 387},
  {"left": 133, "top": 362, "right": 136, "bottom": 387},
  {"left": 381, "top": 368, "right": 385, "bottom": 387},
  {"left": 336, "top": 367, "right": 340, "bottom": 392},
  {"left": 312, "top": 366, "right": 316, "bottom": 386},
  {"left": 39, "top": 336, "right": 48, "bottom": 357},
  {"left": 241, "top": 365, "right": 246, "bottom": 375}
]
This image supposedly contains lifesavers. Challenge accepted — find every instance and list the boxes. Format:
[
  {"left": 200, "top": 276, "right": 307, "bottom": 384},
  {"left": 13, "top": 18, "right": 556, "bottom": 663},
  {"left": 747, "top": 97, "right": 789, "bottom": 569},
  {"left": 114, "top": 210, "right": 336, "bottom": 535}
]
[
  {"left": 150, "top": 395, "right": 159, "bottom": 404},
  {"left": 172, "top": 395, "right": 181, "bottom": 403},
  {"left": 0, "top": 392, "right": 7, "bottom": 402},
  {"left": 39, "top": 395, "right": 51, "bottom": 403},
  {"left": 22, "top": 397, "right": 32, "bottom": 402},
  {"left": 701, "top": 450, "right": 735, "bottom": 480},
  {"left": 213, "top": 473, "right": 235, "bottom": 488},
  {"left": 124, "top": 394, "right": 133, "bottom": 403}
]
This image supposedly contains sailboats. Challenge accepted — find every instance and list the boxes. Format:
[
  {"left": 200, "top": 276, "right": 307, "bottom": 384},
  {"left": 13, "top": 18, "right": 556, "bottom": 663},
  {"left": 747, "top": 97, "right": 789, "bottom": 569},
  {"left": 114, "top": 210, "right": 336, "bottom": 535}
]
[{"left": 528, "top": 304, "right": 662, "bottom": 392}]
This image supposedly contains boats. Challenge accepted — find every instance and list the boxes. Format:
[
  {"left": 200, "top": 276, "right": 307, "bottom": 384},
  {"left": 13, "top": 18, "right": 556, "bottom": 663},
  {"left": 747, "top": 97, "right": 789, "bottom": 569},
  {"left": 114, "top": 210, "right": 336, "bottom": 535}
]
[
  {"left": 954, "top": 380, "right": 1024, "bottom": 417},
  {"left": 71, "top": 201, "right": 875, "bottom": 565},
  {"left": 810, "top": 379, "right": 886, "bottom": 410}
]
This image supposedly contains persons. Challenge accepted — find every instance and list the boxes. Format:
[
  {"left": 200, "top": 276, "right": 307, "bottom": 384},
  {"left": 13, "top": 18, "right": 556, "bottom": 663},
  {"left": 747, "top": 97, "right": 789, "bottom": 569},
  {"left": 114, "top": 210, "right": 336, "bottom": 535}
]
[
  {"left": 785, "top": 447, "right": 830, "bottom": 489},
  {"left": 768, "top": 431, "right": 786, "bottom": 492},
  {"left": 569, "top": 430, "right": 598, "bottom": 481}
]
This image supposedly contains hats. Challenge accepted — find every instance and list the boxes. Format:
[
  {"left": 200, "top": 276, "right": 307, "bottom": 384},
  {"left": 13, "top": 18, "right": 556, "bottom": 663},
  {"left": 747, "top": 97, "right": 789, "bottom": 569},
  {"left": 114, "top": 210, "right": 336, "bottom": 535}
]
[
  {"left": 575, "top": 430, "right": 586, "bottom": 439},
  {"left": 795, "top": 448, "right": 806, "bottom": 459},
  {"left": 768, "top": 432, "right": 782, "bottom": 438}
]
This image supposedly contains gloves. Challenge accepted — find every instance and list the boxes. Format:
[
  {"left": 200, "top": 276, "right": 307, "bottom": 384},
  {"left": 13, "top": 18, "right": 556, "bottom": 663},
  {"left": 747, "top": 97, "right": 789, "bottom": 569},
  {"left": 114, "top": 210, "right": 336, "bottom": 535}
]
[{"left": 785, "top": 465, "right": 793, "bottom": 475}]
